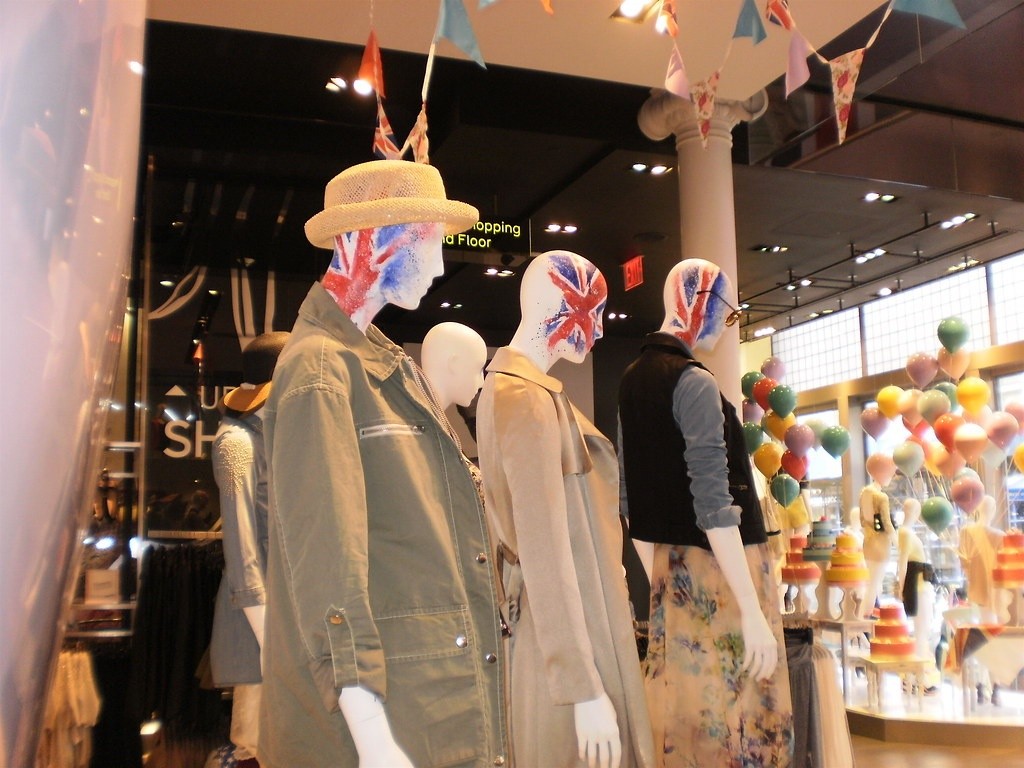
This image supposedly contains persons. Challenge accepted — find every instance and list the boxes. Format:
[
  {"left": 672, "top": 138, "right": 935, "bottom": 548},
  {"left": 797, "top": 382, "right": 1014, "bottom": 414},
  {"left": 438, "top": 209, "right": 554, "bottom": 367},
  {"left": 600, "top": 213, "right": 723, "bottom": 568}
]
[
  {"left": 899, "top": 498, "right": 939, "bottom": 658},
  {"left": 954, "top": 588, "right": 970, "bottom": 609},
  {"left": 857, "top": 452, "right": 897, "bottom": 618},
  {"left": 476, "top": 250, "right": 655, "bottom": 768},
  {"left": 209, "top": 331, "right": 292, "bottom": 768},
  {"left": 777, "top": 489, "right": 813, "bottom": 539},
  {"left": 421, "top": 320, "right": 482, "bottom": 511},
  {"left": 976, "top": 496, "right": 1007, "bottom": 547},
  {"left": 617, "top": 258, "right": 795, "bottom": 768},
  {"left": 256, "top": 158, "right": 507, "bottom": 768}
]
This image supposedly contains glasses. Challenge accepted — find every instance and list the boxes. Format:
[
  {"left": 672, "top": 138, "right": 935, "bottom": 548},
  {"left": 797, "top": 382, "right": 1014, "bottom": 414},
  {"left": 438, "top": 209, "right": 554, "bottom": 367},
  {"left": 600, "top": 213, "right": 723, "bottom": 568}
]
[{"left": 697, "top": 291, "right": 743, "bottom": 327}]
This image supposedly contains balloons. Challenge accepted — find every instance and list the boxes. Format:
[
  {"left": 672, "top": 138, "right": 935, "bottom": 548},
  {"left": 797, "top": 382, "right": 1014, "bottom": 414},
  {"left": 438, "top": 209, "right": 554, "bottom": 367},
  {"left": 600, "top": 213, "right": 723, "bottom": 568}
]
[
  {"left": 740, "top": 356, "right": 824, "bottom": 509},
  {"left": 821, "top": 426, "right": 851, "bottom": 459},
  {"left": 861, "top": 318, "right": 1024, "bottom": 536}
]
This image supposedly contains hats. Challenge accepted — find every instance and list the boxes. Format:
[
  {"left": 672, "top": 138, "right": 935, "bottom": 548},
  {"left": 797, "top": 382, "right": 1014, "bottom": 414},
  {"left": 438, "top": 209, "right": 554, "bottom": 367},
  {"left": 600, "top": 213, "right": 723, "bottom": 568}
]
[
  {"left": 217, "top": 331, "right": 291, "bottom": 418},
  {"left": 304, "top": 160, "right": 479, "bottom": 250}
]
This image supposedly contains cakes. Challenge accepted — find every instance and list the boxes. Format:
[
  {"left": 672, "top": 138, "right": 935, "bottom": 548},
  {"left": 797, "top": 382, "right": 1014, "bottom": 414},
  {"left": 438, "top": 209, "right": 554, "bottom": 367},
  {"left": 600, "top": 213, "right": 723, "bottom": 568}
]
[{"left": 782, "top": 515, "right": 1024, "bottom": 663}]
